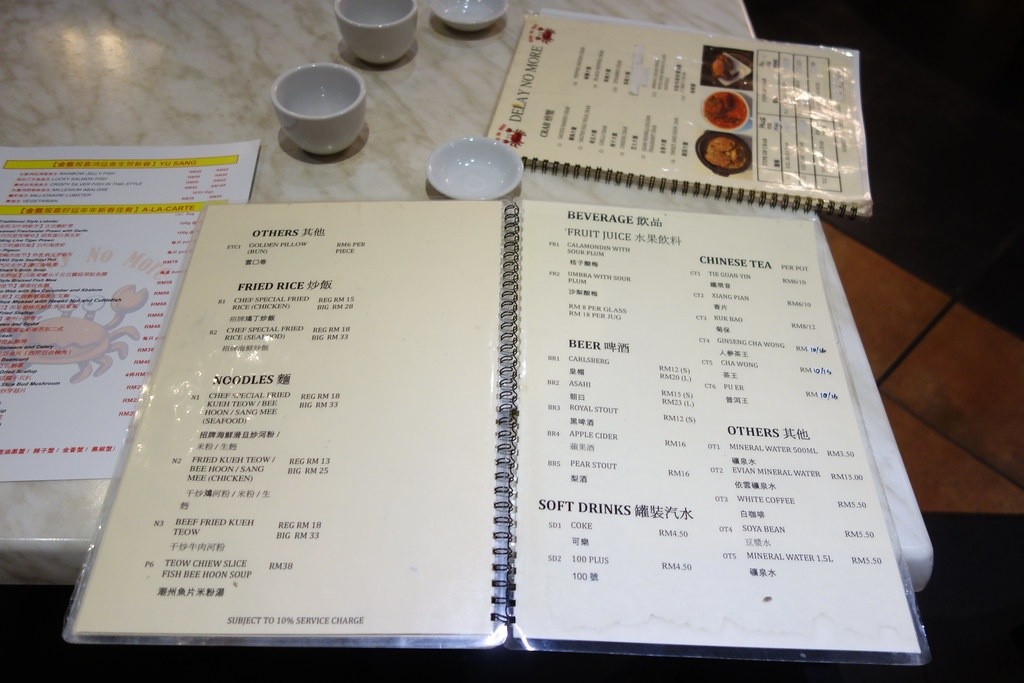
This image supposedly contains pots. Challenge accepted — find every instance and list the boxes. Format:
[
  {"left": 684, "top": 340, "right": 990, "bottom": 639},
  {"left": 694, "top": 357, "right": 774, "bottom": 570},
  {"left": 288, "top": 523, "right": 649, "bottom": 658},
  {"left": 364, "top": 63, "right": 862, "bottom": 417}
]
[{"left": 696, "top": 130, "right": 751, "bottom": 177}]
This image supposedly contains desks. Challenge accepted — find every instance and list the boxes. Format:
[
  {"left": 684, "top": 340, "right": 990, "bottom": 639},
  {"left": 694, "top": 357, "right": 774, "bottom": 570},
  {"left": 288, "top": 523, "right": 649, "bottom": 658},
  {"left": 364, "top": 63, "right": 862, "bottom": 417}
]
[{"left": 0, "top": 0, "right": 936, "bottom": 595}]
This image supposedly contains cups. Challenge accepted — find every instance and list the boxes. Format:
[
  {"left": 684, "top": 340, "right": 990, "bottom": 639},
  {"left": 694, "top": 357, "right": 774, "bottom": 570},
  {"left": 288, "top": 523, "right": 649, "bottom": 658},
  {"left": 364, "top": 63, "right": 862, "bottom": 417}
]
[
  {"left": 335, "top": 0, "right": 419, "bottom": 66},
  {"left": 269, "top": 62, "right": 367, "bottom": 157}
]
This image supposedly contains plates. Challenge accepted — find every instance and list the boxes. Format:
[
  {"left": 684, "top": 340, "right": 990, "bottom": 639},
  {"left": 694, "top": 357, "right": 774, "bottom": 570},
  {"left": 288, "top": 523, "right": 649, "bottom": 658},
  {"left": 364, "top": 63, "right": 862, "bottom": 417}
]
[
  {"left": 428, "top": 0, "right": 509, "bottom": 32},
  {"left": 698, "top": 90, "right": 751, "bottom": 132},
  {"left": 426, "top": 136, "right": 524, "bottom": 201},
  {"left": 718, "top": 52, "right": 751, "bottom": 86}
]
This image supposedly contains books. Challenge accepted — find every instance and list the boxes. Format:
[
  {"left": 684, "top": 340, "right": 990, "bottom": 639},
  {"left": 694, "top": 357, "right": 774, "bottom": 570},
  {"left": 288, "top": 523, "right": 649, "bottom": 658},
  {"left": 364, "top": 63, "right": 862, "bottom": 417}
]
[
  {"left": 484, "top": 7, "right": 880, "bottom": 220},
  {"left": 63, "top": 193, "right": 933, "bottom": 672}
]
